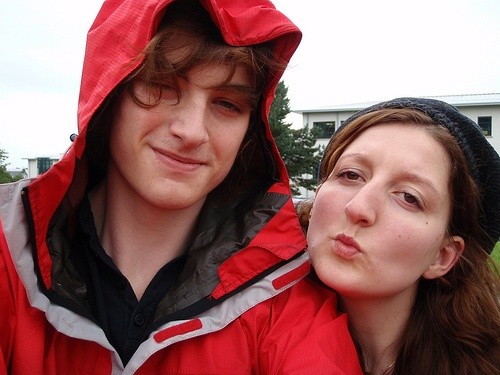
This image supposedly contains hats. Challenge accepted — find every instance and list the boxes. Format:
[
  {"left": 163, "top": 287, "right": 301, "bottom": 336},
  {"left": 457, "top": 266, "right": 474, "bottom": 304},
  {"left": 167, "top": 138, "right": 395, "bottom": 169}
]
[{"left": 315, "top": 98, "right": 500, "bottom": 257}]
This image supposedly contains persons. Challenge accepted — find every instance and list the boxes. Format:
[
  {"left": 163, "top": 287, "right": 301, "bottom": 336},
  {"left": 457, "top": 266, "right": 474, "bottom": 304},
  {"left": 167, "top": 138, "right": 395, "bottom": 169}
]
[
  {"left": 0, "top": 0, "right": 364, "bottom": 375},
  {"left": 295, "top": 98, "right": 500, "bottom": 375}
]
